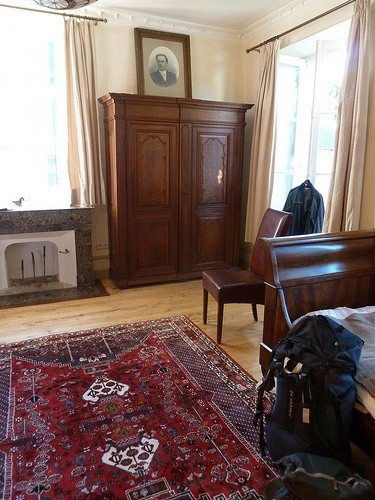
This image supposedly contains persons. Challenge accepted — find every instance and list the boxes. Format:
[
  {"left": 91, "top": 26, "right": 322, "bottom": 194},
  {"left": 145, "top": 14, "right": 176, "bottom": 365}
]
[{"left": 149, "top": 53, "right": 178, "bottom": 88}]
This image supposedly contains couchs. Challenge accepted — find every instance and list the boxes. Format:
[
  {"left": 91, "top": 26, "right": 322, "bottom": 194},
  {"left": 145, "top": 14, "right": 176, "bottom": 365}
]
[{"left": 256, "top": 230, "right": 375, "bottom": 418}]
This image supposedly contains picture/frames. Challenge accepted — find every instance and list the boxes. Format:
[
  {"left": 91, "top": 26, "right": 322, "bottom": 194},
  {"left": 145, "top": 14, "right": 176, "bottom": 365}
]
[{"left": 134, "top": 27, "right": 192, "bottom": 99}]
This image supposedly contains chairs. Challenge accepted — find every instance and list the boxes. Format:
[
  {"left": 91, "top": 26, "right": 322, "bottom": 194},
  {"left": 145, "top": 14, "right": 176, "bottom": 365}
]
[{"left": 202, "top": 208, "right": 294, "bottom": 344}]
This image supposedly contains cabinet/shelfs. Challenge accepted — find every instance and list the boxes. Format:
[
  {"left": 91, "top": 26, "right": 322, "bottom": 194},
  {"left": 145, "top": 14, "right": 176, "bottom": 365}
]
[{"left": 97, "top": 92, "right": 255, "bottom": 289}]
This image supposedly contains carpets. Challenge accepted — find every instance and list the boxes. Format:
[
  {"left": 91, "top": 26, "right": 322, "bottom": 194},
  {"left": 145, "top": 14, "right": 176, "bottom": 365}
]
[{"left": 0, "top": 314, "right": 281, "bottom": 500}]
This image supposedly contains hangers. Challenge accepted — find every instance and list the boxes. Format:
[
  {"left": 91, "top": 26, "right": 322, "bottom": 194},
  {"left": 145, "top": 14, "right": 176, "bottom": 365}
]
[{"left": 302, "top": 179, "right": 311, "bottom": 192}]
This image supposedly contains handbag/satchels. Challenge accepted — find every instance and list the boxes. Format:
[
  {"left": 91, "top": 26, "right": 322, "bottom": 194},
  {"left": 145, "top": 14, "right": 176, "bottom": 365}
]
[{"left": 263, "top": 454, "right": 374, "bottom": 500}]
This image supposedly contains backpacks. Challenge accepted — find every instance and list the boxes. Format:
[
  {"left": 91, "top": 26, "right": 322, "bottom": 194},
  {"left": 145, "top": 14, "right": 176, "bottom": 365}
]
[{"left": 251, "top": 314, "right": 364, "bottom": 462}]
